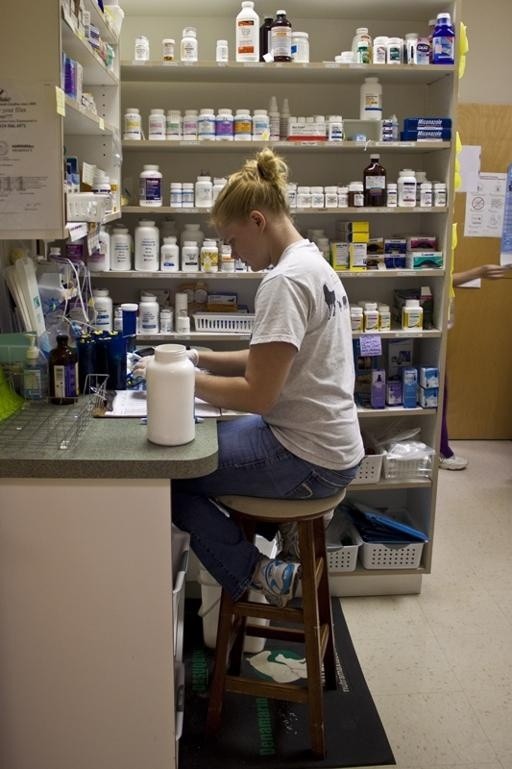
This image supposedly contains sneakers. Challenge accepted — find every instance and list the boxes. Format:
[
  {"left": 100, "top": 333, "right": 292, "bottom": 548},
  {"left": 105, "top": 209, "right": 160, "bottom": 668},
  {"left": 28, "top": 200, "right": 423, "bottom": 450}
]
[
  {"left": 438, "top": 454, "right": 467, "bottom": 470},
  {"left": 250, "top": 556, "right": 301, "bottom": 609},
  {"left": 278, "top": 509, "right": 335, "bottom": 559}
]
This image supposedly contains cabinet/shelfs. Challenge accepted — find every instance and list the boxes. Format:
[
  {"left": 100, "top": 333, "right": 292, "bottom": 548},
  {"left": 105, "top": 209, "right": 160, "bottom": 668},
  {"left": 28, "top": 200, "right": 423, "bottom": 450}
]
[
  {"left": 2, "top": 0, "right": 122, "bottom": 243},
  {"left": 38, "top": 0, "right": 459, "bottom": 599}
]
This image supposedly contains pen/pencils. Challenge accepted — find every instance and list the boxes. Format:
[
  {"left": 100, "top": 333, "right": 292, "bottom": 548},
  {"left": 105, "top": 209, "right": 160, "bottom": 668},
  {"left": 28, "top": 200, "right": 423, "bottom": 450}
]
[{"left": 142, "top": 417, "right": 206, "bottom": 424}]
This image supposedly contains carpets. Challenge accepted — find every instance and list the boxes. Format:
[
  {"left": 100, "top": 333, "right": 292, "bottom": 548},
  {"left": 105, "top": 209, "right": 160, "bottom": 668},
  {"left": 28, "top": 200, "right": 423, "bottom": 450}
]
[{"left": 179, "top": 591, "right": 400, "bottom": 768}]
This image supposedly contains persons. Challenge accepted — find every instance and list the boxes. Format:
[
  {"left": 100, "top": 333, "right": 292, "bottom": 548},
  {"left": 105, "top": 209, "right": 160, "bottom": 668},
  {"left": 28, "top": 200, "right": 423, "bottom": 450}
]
[
  {"left": 438, "top": 262, "right": 507, "bottom": 472},
  {"left": 129, "top": 145, "right": 367, "bottom": 610}
]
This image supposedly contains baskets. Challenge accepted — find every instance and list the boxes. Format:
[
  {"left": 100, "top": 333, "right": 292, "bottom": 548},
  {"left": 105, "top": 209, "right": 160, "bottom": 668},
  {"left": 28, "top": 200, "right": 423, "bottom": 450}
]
[
  {"left": 194, "top": 312, "right": 255, "bottom": 333},
  {"left": 361, "top": 508, "right": 425, "bottom": 569},
  {"left": 384, "top": 453, "right": 430, "bottom": 480},
  {"left": 0, "top": 374, "right": 108, "bottom": 449},
  {"left": 322, "top": 512, "right": 362, "bottom": 572},
  {"left": 349, "top": 438, "right": 386, "bottom": 483}
]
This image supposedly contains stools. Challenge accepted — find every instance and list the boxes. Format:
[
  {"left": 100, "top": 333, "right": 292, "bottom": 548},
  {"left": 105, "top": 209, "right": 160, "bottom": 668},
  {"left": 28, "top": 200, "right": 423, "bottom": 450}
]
[{"left": 206, "top": 487, "right": 347, "bottom": 759}]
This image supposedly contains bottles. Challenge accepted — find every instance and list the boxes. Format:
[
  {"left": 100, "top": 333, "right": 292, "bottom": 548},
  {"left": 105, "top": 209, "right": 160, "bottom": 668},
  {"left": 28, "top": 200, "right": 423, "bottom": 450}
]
[
  {"left": 159, "top": 309, "right": 173, "bottom": 333},
  {"left": 161, "top": 237, "right": 179, "bottom": 272},
  {"left": 147, "top": 108, "right": 272, "bottom": 141},
  {"left": 308, "top": 228, "right": 330, "bottom": 263},
  {"left": 349, "top": 301, "right": 391, "bottom": 332},
  {"left": 216, "top": 40, "right": 228, "bottom": 62},
  {"left": 91, "top": 176, "right": 111, "bottom": 213},
  {"left": 134, "top": 217, "right": 159, "bottom": 272},
  {"left": 352, "top": 27, "right": 373, "bottom": 64},
  {"left": 348, "top": 180, "right": 364, "bottom": 206},
  {"left": 236, "top": 1, "right": 259, "bottom": 63},
  {"left": 269, "top": 96, "right": 281, "bottom": 140},
  {"left": 145, "top": 344, "right": 196, "bottom": 446},
  {"left": 373, "top": 36, "right": 403, "bottom": 64},
  {"left": 213, "top": 177, "right": 226, "bottom": 206},
  {"left": 281, "top": 98, "right": 290, "bottom": 137},
  {"left": 182, "top": 241, "right": 199, "bottom": 271},
  {"left": 259, "top": 16, "right": 275, "bottom": 63},
  {"left": 162, "top": 39, "right": 175, "bottom": 61},
  {"left": 402, "top": 299, "right": 424, "bottom": 330},
  {"left": 123, "top": 108, "right": 141, "bottom": 140},
  {"left": 181, "top": 28, "right": 198, "bottom": 61},
  {"left": 181, "top": 224, "right": 204, "bottom": 240},
  {"left": 139, "top": 296, "right": 158, "bottom": 333},
  {"left": 92, "top": 289, "right": 112, "bottom": 333},
  {"left": 49, "top": 335, "right": 79, "bottom": 405},
  {"left": 271, "top": 10, "right": 292, "bottom": 62},
  {"left": 329, "top": 116, "right": 342, "bottom": 141},
  {"left": 182, "top": 182, "right": 194, "bottom": 207},
  {"left": 292, "top": 31, "right": 309, "bottom": 63},
  {"left": 134, "top": 37, "right": 149, "bottom": 60},
  {"left": 364, "top": 153, "right": 386, "bottom": 206},
  {"left": 403, "top": 33, "right": 418, "bottom": 64},
  {"left": 140, "top": 165, "right": 162, "bottom": 207},
  {"left": 387, "top": 169, "right": 446, "bottom": 208},
  {"left": 287, "top": 184, "right": 349, "bottom": 208},
  {"left": 170, "top": 183, "right": 182, "bottom": 208},
  {"left": 110, "top": 226, "right": 131, "bottom": 270},
  {"left": 195, "top": 176, "right": 212, "bottom": 207},
  {"left": 433, "top": 13, "right": 455, "bottom": 63},
  {"left": 175, "top": 293, "right": 187, "bottom": 317},
  {"left": 359, "top": 77, "right": 382, "bottom": 119},
  {"left": 201, "top": 241, "right": 219, "bottom": 272}
]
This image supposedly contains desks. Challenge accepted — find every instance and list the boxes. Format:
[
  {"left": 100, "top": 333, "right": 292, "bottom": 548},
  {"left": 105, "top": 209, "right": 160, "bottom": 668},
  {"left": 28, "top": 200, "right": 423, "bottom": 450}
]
[{"left": 0, "top": 400, "right": 221, "bottom": 769}]
